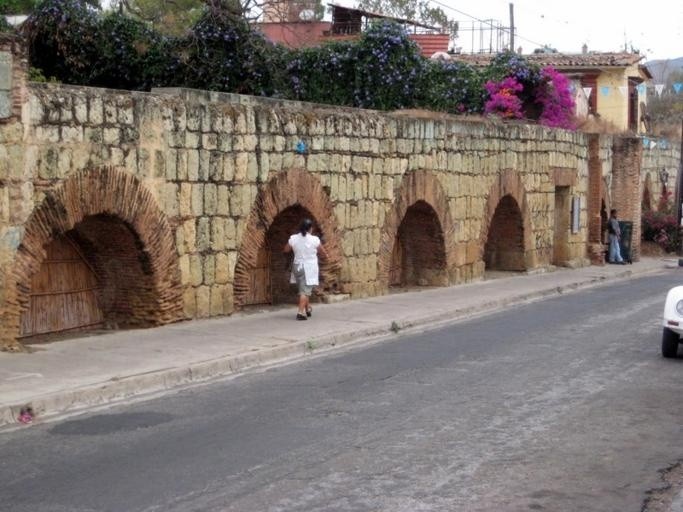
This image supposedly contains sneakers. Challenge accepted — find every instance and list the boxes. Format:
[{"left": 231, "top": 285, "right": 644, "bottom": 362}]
[
  {"left": 610, "top": 261, "right": 626, "bottom": 264},
  {"left": 297, "top": 311, "right": 310, "bottom": 320}
]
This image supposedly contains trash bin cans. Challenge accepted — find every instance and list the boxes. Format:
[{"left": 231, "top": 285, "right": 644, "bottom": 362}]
[{"left": 618, "top": 221, "right": 633, "bottom": 264}]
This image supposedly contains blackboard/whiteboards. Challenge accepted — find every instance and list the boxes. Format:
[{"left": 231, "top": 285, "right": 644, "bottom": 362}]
[{"left": 572, "top": 196, "right": 580, "bottom": 234}]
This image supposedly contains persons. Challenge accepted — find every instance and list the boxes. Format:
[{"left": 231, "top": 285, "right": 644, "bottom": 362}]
[
  {"left": 281, "top": 218, "right": 330, "bottom": 320},
  {"left": 607, "top": 209, "right": 627, "bottom": 265}
]
